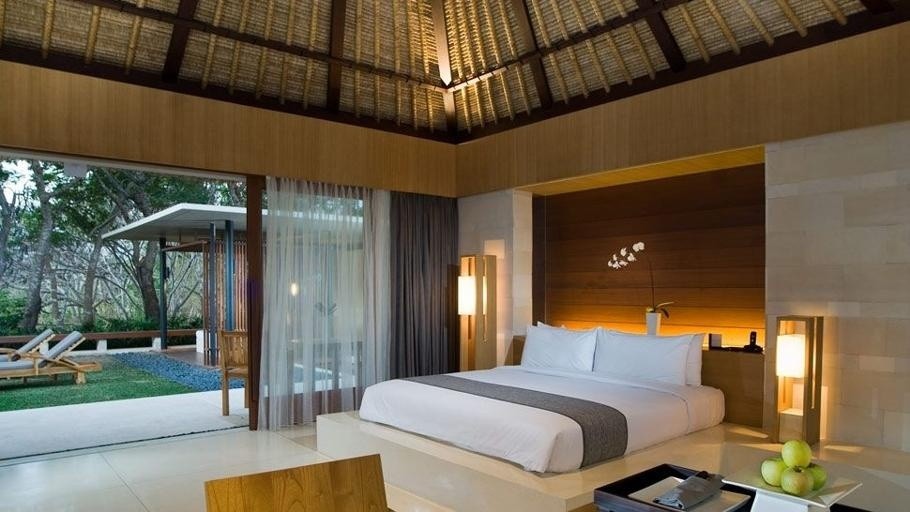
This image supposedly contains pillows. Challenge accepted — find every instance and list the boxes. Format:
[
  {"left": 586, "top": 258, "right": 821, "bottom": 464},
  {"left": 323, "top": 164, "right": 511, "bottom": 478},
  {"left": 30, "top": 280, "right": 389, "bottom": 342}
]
[{"left": 515, "top": 321, "right": 706, "bottom": 389}]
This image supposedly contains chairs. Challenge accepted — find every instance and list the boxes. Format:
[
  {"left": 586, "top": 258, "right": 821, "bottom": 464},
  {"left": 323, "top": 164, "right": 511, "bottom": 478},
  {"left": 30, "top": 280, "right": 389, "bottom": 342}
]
[{"left": 1, "top": 324, "right": 107, "bottom": 387}]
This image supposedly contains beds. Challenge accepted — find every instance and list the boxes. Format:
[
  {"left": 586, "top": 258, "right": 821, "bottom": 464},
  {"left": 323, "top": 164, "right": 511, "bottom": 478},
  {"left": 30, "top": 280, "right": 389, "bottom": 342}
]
[{"left": 356, "top": 363, "right": 729, "bottom": 480}]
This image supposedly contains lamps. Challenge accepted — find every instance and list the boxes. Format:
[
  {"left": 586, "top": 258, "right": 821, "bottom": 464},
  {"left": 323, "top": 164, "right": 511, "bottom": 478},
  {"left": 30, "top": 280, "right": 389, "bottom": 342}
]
[
  {"left": 454, "top": 253, "right": 499, "bottom": 371},
  {"left": 770, "top": 312, "right": 826, "bottom": 448}
]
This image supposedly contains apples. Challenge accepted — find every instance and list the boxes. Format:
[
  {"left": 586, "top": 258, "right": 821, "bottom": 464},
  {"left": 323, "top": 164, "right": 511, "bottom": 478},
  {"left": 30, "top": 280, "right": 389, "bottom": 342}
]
[
  {"left": 806, "top": 463, "right": 828, "bottom": 491},
  {"left": 761, "top": 457, "right": 788, "bottom": 487},
  {"left": 781, "top": 465, "right": 814, "bottom": 496},
  {"left": 781, "top": 439, "right": 812, "bottom": 469}
]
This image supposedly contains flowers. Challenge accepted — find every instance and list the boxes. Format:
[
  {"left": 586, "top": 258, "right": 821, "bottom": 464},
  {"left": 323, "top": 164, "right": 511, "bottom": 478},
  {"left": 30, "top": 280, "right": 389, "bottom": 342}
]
[{"left": 608, "top": 239, "right": 678, "bottom": 320}]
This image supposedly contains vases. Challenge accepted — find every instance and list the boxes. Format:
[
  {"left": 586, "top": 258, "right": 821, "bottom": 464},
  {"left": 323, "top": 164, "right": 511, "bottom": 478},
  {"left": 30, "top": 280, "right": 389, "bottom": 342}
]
[{"left": 645, "top": 309, "right": 662, "bottom": 334}]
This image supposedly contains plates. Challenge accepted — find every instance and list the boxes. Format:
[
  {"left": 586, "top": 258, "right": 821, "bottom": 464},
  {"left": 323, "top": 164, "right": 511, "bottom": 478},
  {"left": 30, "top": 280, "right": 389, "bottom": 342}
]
[
  {"left": 721, "top": 456, "right": 864, "bottom": 508},
  {"left": 628, "top": 475, "right": 751, "bottom": 512}
]
